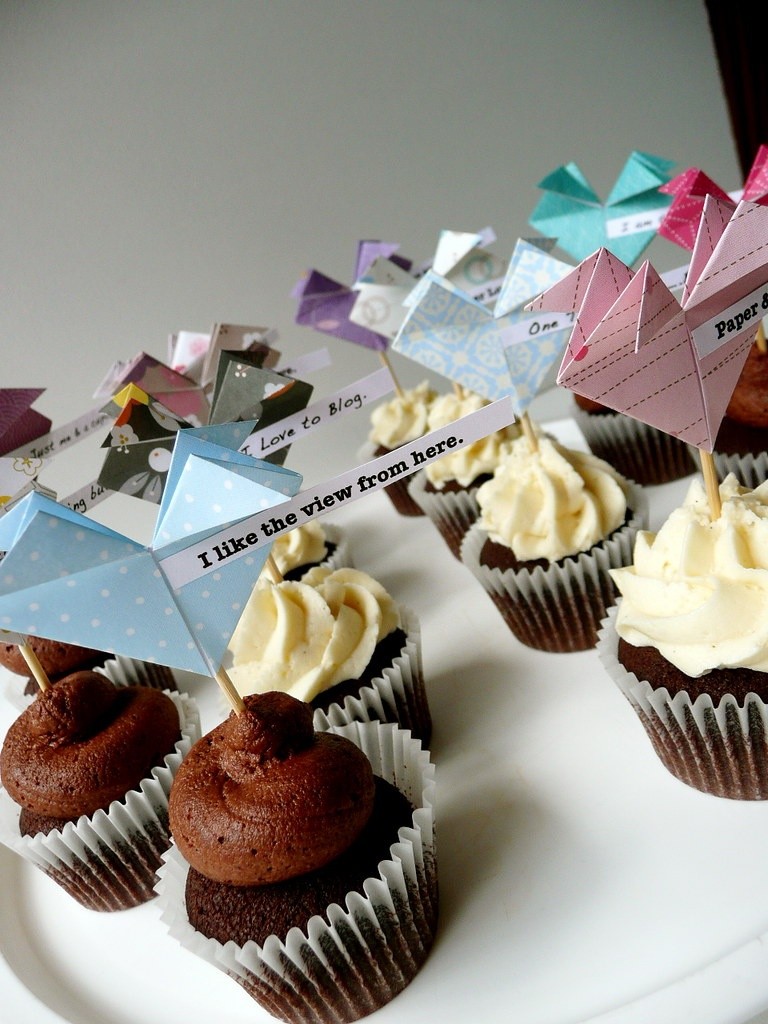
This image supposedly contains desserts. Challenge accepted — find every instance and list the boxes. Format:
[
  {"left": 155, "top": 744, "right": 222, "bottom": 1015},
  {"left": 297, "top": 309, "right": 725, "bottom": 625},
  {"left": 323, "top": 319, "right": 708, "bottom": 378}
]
[
  {"left": 0, "top": 488, "right": 442, "bottom": 1024},
  {"left": 366, "top": 316, "right": 768, "bottom": 800}
]
